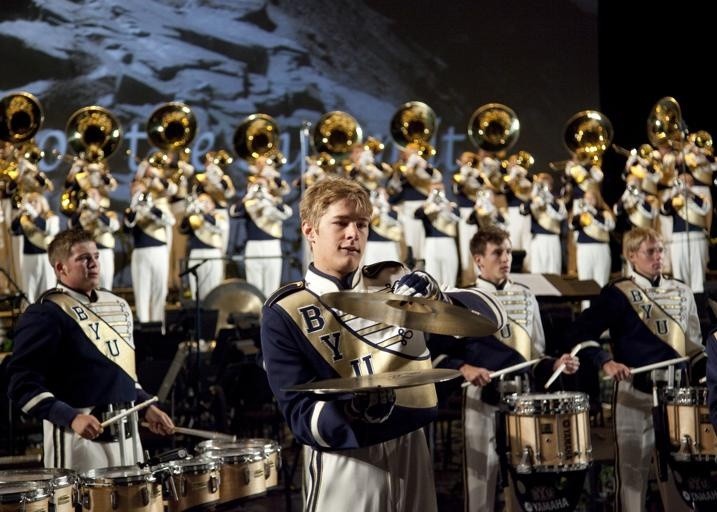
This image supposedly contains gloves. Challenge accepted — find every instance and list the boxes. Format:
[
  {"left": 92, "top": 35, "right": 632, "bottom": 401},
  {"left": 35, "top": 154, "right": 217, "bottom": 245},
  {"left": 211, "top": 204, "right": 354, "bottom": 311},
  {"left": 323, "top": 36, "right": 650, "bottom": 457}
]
[
  {"left": 344, "top": 390, "right": 396, "bottom": 424},
  {"left": 393, "top": 270, "right": 454, "bottom": 306}
]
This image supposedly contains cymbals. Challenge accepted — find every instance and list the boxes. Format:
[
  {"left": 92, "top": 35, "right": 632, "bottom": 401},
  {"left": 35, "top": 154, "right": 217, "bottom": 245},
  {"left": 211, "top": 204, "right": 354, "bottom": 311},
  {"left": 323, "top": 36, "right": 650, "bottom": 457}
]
[
  {"left": 277, "top": 368, "right": 461, "bottom": 393},
  {"left": 319, "top": 292, "right": 497, "bottom": 338}
]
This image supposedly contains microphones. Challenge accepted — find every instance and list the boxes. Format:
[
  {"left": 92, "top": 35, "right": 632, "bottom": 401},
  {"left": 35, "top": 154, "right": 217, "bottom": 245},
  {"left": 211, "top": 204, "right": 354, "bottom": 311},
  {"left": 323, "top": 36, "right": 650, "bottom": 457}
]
[{"left": 178, "top": 260, "right": 207, "bottom": 277}]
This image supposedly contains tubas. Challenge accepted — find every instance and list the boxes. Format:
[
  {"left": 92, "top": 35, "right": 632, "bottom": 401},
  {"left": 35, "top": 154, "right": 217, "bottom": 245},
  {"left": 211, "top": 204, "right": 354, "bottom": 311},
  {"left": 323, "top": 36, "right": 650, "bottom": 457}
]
[
  {"left": 648, "top": 96, "right": 690, "bottom": 163},
  {"left": 468, "top": 103, "right": 521, "bottom": 168},
  {"left": 389, "top": 100, "right": 439, "bottom": 178},
  {"left": 308, "top": 111, "right": 363, "bottom": 173},
  {"left": 560, "top": 109, "right": 613, "bottom": 173},
  {"left": 231, "top": 113, "right": 286, "bottom": 175},
  {"left": 148, "top": 100, "right": 198, "bottom": 180},
  {"left": 0, "top": 89, "right": 45, "bottom": 186},
  {"left": 67, "top": 106, "right": 121, "bottom": 186}
]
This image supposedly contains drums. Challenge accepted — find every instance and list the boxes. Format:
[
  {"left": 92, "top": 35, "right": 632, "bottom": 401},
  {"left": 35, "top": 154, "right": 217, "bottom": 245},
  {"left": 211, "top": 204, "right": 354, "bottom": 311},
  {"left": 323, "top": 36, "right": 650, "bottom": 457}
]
[
  {"left": 152, "top": 454, "right": 224, "bottom": 511},
  {"left": 78, "top": 464, "right": 164, "bottom": 511},
  {"left": 195, "top": 436, "right": 281, "bottom": 502},
  {"left": 1, "top": 467, "right": 80, "bottom": 512},
  {"left": 666, "top": 386, "right": 717, "bottom": 462},
  {"left": 503, "top": 391, "right": 594, "bottom": 474}
]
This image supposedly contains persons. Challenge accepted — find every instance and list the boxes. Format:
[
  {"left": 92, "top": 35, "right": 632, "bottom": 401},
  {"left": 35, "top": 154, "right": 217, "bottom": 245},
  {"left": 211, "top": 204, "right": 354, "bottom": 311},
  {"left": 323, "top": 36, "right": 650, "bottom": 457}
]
[{"left": 1, "top": 141, "right": 716, "bottom": 512}]
